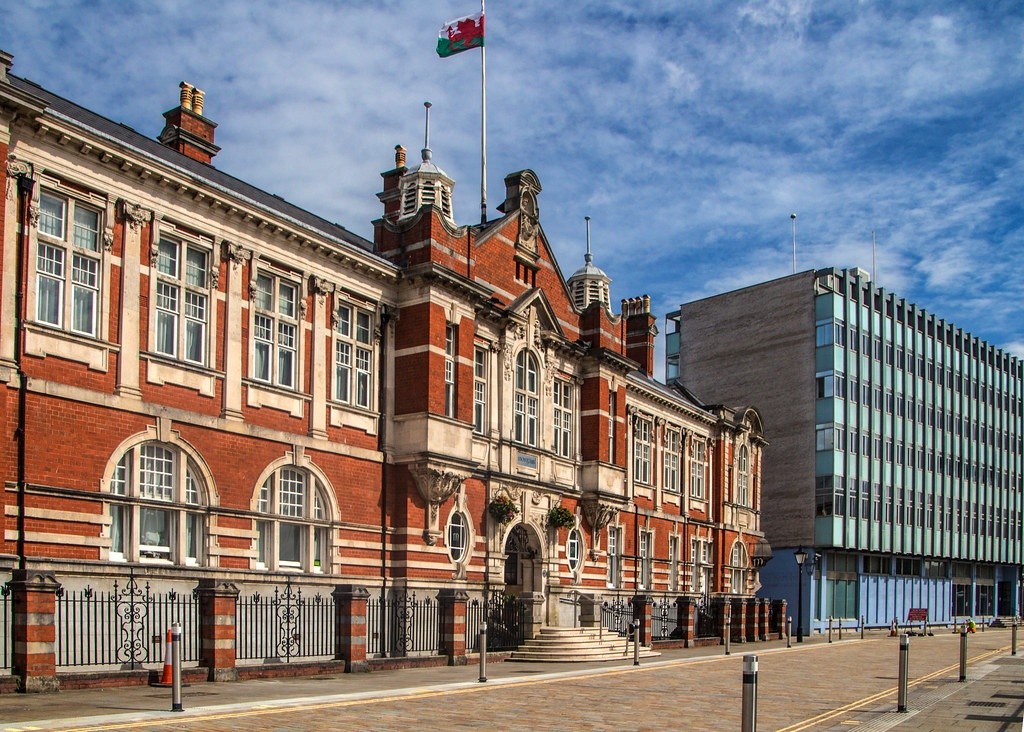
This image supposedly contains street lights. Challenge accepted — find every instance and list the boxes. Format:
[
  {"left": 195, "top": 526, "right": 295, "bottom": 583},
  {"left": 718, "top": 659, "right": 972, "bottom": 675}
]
[{"left": 793, "top": 543, "right": 807, "bottom": 643}]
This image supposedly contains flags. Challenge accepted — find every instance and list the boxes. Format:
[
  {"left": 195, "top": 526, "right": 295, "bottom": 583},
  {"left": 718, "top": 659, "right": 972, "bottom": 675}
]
[{"left": 436, "top": 10, "right": 486, "bottom": 58}]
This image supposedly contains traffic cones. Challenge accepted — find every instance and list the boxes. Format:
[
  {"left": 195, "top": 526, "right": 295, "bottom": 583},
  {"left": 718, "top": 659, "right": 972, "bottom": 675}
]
[
  {"left": 150, "top": 629, "right": 191, "bottom": 688},
  {"left": 888, "top": 619, "right": 897, "bottom": 637}
]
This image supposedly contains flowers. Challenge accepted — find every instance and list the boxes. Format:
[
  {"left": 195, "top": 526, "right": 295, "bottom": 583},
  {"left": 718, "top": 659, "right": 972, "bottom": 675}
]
[
  {"left": 488, "top": 494, "right": 520, "bottom": 526},
  {"left": 545, "top": 504, "right": 575, "bottom": 531}
]
